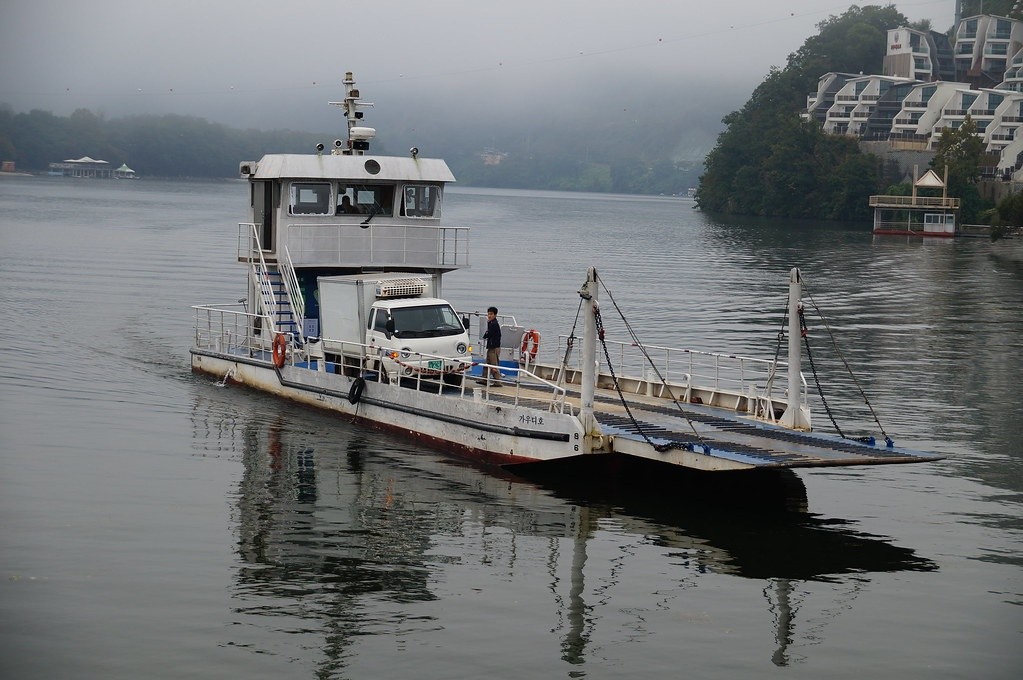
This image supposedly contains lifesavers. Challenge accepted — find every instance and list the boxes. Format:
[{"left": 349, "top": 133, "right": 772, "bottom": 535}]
[
  {"left": 347, "top": 377, "right": 366, "bottom": 406},
  {"left": 522, "top": 329, "right": 539, "bottom": 363},
  {"left": 273, "top": 334, "right": 287, "bottom": 369}
]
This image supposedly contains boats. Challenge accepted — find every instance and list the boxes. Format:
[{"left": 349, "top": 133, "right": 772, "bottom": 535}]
[{"left": 189, "top": 70, "right": 947, "bottom": 478}]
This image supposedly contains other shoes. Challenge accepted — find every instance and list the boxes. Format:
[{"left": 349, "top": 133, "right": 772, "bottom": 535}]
[
  {"left": 490, "top": 383, "right": 503, "bottom": 387},
  {"left": 476, "top": 380, "right": 487, "bottom": 386}
]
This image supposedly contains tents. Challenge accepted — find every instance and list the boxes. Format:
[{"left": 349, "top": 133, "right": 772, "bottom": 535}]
[{"left": 116, "top": 163, "right": 135, "bottom": 177}]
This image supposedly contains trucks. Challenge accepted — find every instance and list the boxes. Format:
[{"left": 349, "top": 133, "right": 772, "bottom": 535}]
[{"left": 316, "top": 271, "right": 472, "bottom": 390}]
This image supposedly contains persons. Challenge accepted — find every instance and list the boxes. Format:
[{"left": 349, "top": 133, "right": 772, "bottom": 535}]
[
  {"left": 335, "top": 195, "right": 361, "bottom": 214},
  {"left": 474, "top": 307, "right": 504, "bottom": 387}
]
